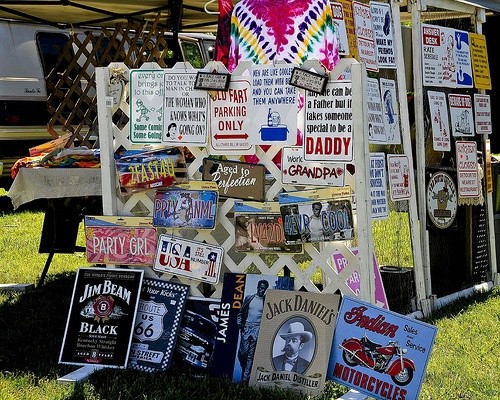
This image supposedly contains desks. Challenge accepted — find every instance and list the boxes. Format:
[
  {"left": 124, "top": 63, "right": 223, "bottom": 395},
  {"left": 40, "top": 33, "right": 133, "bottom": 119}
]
[{"left": 6, "top": 167, "right": 103, "bottom": 291}]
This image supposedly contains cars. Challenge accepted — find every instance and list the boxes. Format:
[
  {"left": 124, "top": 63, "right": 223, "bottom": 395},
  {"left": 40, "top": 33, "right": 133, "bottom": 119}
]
[{"left": 174, "top": 309, "right": 218, "bottom": 369}]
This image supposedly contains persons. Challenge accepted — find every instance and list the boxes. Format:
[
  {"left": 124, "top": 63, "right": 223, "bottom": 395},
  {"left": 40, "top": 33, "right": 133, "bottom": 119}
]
[
  {"left": 232, "top": 277, "right": 277, "bottom": 387},
  {"left": 307, "top": 202, "right": 327, "bottom": 242},
  {"left": 274, "top": 321, "right": 312, "bottom": 376}
]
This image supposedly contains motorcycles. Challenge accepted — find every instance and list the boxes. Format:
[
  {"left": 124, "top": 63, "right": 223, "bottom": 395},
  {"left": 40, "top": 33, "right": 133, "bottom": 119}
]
[{"left": 339, "top": 333, "right": 416, "bottom": 386}]
